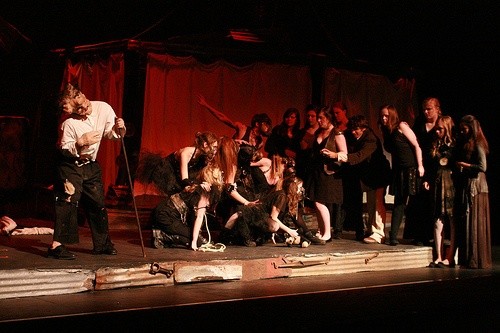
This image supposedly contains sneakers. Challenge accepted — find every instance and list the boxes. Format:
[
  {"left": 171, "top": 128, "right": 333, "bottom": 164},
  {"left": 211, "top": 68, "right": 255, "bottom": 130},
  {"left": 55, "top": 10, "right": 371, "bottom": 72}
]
[
  {"left": 151, "top": 228, "right": 164, "bottom": 249},
  {"left": 363, "top": 234, "right": 385, "bottom": 243}
]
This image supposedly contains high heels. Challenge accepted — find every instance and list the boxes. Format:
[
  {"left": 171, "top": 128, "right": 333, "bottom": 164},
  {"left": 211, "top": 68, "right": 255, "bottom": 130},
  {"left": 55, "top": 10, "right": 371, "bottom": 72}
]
[{"left": 320, "top": 236, "right": 332, "bottom": 242}]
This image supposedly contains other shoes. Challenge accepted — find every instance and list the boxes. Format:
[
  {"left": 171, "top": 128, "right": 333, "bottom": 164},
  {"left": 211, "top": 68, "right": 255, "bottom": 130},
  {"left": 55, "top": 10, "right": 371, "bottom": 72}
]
[
  {"left": 216, "top": 227, "right": 232, "bottom": 248},
  {"left": 243, "top": 238, "right": 258, "bottom": 247},
  {"left": 429, "top": 259, "right": 455, "bottom": 268},
  {"left": 389, "top": 238, "right": 399, "bottom": 245},
  {"left": 404, "top": 236, "right": 418, "bottom": 244}
]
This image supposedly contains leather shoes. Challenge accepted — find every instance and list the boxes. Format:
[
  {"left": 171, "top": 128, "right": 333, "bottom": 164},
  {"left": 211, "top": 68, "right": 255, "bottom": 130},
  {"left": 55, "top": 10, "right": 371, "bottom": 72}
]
[
  {"left": 47, "top": 245, "right": 76, "bottom": 260},
  {"left": 92, "top": 247, "right": 117, "bottom": 255}
]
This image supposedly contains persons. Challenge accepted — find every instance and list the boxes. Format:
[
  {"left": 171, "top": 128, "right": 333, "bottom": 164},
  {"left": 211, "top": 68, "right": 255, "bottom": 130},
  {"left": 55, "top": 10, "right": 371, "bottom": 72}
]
[
  {"left": 290, "top": 105, "right": 321, "bottom": 198},
  {"left": 329, "top": 114, "right": 392, "bottom": 243},
  {"left": 331, "top": 102, "right": 365, "bottom": 242},
  {"left": 403, "top": 98, "right": 442, "bottom": 247},
  {"left": 137, "top": 134, "right": 325, "bottom": 253},
  {"left": 46, "top": 83, "right": 126, "bottom": 259},
  {"left": 195, "top": 93, "right": 272, "bottom": 149},
  {"left": 264, "top": 107, "right": 300, "bottom": 160},
  {"left": 423, "top": 116, "right": 462, "bottom": 269},
  {"left": 456, "top": 115, "right": 492, "bottom": 269},
  {"left": 311, "top": 105, "right": 348, "bottom": 243},
  {"left": 377, "top": 103, "right": 425, "bottom": 245}
]
